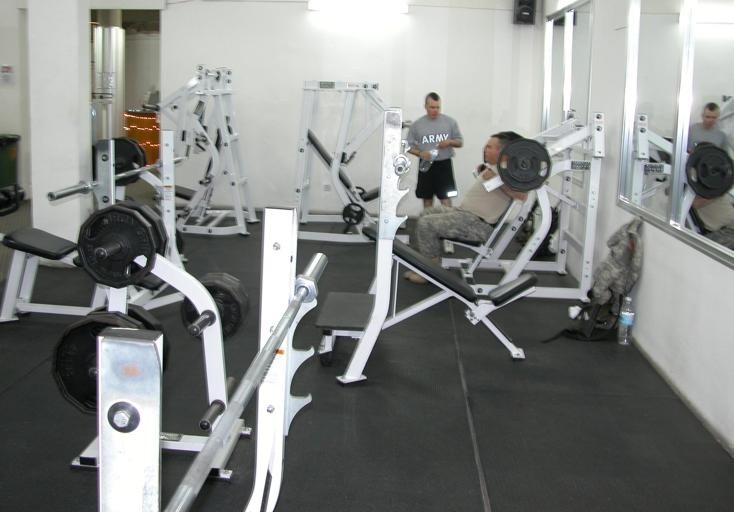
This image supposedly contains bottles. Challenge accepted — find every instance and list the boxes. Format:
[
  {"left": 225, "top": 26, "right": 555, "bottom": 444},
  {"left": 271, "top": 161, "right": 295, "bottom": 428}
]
[
  {"left": 419, "top": 144, "right": 440, "bottom": 173},
  {"left": 618, "top": 296, "right": 636, "bottom": 346}
]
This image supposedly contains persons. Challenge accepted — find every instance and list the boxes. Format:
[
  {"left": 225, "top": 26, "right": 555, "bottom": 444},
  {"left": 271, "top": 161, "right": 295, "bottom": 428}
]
[
  {"left": 402, "top": 129, "right": 529, "bottom": 284},
  {"left": 406, "top": 91, "right": 465, "bottom": 254},
  {"left": 683, "top": 141, "right": 734, "bottom": 252},
  {"left": 686, "top": 99, "right": 729, "bottom": 159}
]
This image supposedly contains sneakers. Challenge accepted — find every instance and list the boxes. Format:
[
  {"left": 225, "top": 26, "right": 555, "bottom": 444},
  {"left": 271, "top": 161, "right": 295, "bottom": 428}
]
[
  {"left": 410, "top": 273, "right": 430, "bottom": 284},
  {"left": 403, "top": 270, "right": 415, "bottom": 278}
]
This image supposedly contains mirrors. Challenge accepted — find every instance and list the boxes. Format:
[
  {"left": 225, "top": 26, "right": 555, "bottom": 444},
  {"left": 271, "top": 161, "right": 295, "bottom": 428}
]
[
  {"left": 617, "top": 0, "right": 734, "bottom": 267},
  {"left": 541, "top": 0, "right": 597, "bottom": 154}
]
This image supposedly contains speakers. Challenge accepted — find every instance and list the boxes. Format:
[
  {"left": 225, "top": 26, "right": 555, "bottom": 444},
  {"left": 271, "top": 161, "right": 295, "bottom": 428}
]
[{"left": 513, "top": 0, "right": 535, "bottom": 25}]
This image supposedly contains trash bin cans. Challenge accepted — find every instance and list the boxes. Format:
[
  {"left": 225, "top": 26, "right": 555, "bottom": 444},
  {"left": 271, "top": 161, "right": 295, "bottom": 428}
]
[{"left": 0, "top": 134, "right": 21, "bottom": 188}]
